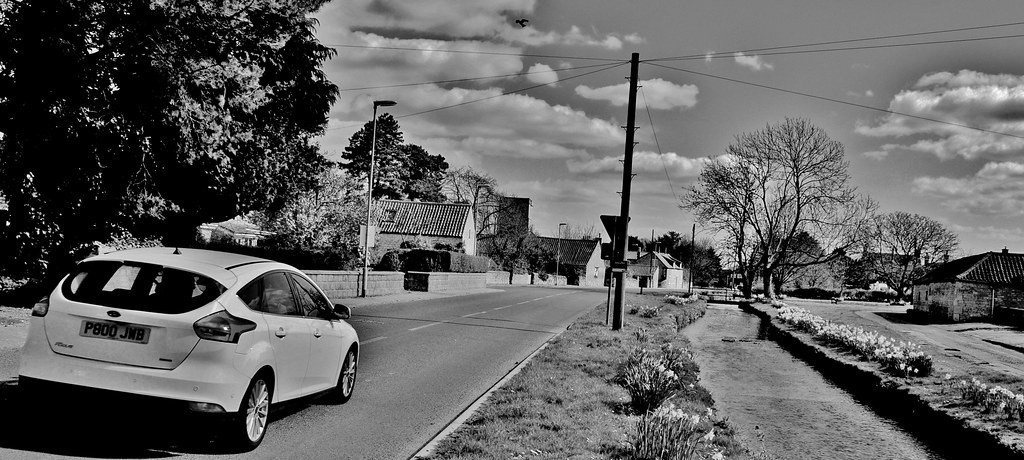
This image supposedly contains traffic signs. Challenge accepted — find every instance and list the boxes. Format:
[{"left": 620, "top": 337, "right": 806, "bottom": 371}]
[{"left": 611, "top": 260, "right": 627, "bottom": 273}]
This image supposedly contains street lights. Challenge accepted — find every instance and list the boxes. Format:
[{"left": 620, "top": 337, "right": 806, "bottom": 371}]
[
  {"left": 554, "top": 223, "right": 567, "bottom": 286},
  {"left": 655, "top": 240, "right": 662, "bottom": 289},
  {"left": 362, "top": 99, "right": 397, "bottom": 296}
]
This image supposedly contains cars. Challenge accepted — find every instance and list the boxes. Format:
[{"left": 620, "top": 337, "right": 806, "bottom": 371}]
[{"left": 15, "top": 247, "right": 363, "bottom": 452}]
